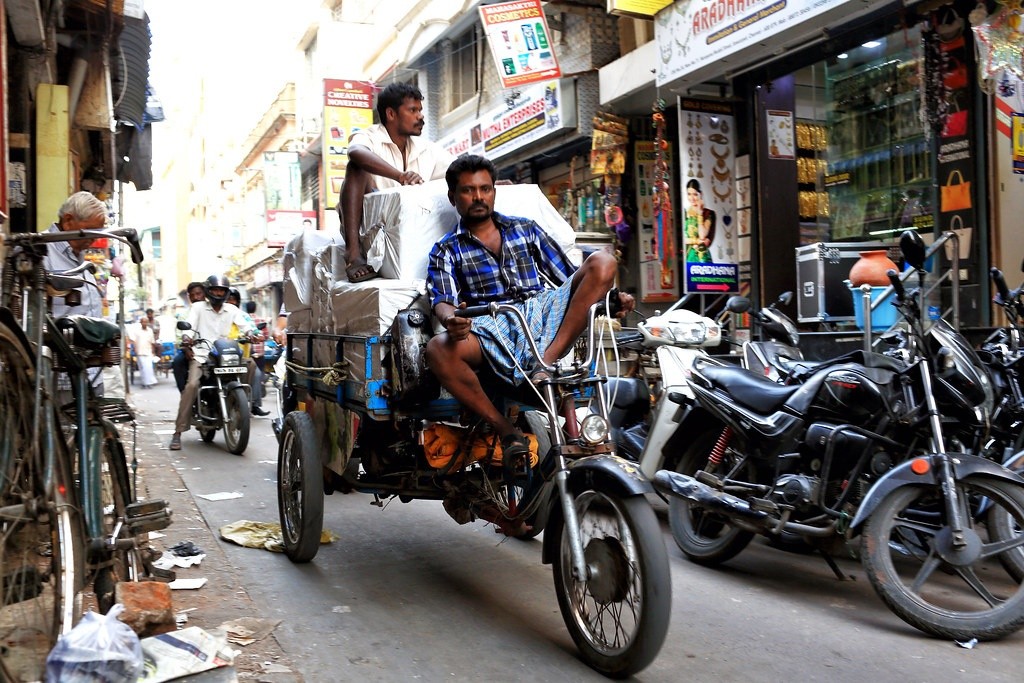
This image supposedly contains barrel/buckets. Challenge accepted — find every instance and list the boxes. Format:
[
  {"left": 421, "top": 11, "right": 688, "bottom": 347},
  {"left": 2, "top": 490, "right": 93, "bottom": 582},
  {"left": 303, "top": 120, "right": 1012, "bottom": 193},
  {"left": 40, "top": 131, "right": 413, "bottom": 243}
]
[
  {"left": 594, "top": 333, "right": 627, "bottom": 375},
  {"left": 843, "top": 279, "right": 901, "bottom": 332}
]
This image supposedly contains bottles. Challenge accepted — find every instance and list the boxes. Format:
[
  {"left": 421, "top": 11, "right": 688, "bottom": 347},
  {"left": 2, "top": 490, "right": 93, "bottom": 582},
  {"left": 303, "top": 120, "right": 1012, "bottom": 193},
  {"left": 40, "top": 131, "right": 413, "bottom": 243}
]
[{"left": 848, "top": 250, "right": 898, "bottom": 286}]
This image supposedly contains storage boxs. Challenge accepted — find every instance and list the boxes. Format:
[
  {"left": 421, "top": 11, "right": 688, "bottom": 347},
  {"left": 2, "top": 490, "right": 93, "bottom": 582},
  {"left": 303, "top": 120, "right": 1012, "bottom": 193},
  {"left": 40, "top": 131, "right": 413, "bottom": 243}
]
[{"left": 796, "top": 222, "right": 905, "bottom": 321}]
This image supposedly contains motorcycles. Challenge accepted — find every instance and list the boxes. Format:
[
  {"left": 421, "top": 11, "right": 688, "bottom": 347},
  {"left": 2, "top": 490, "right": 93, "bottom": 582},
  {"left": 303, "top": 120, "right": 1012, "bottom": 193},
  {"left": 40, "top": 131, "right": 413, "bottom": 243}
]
[
  {"left": 123, "top": 297, "right": 285, "bottom": 456},
  {"left": 269, "top": 245, "right": 673, "bottom": 682},
  {"left": 589, "top": 226, "right": 1024, "bottom": 644}
]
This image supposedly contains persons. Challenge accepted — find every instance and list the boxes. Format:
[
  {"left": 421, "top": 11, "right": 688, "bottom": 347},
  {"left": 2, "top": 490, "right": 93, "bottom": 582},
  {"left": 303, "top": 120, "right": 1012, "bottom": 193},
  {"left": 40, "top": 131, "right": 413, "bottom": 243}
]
[
  {"left": 686, "top": 179, "right": 716, "bottom": 263},
  {"left": 424, "top": 154, "right": 636, "bottom": 470},
  {"left": 135, "top": 309, "right": 160, "bottom": 388},
  {"left": 170, "top": 274, "right": 270, "bottom": 450},
  {"left": 337, "top": 82, "right": 454, "bottom": 283},
  {"left": 14, "top": 191, "right": 104, "bottom": 555}
]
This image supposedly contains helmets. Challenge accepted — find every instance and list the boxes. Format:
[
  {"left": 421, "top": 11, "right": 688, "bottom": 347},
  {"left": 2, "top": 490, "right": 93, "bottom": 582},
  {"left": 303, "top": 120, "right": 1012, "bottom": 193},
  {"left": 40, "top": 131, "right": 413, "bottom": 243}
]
[
  {"left": 229, "top": 287, "right": 241, "bottom": 308},
  {"left": 203, "top": 274, "right": 229, "bottom": 304}
]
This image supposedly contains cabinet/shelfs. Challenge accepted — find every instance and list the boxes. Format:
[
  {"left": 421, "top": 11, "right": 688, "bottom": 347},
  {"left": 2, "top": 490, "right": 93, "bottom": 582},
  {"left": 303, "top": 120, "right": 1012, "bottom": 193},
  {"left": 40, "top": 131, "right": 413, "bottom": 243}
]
[{"left": 796, "top": 55, "right": 932, "bottom": 242}]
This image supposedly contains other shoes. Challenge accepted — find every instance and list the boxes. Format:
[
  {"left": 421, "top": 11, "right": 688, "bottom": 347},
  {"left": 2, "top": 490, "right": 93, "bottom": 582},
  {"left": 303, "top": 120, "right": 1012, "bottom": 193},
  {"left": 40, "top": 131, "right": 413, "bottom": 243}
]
[
  {"left": 251, "top": 407, "right": 271, "bottom": 415},
  {"left": 169, "top": 432, "right": 181, "bottom": 450}
]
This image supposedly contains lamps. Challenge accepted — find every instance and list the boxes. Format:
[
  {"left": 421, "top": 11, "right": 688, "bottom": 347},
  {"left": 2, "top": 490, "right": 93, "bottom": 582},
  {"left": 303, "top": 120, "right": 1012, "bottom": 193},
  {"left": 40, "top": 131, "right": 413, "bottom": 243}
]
[{"left": 724, "top": 27, "right": 831, "bottom": 81}]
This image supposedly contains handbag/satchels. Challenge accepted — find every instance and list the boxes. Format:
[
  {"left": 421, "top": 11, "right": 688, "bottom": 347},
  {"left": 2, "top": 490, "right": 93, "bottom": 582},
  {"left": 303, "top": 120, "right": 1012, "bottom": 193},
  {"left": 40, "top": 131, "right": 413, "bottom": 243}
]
[
  {"left": 937, "top": 9, "right": 966, "bottom": 52},
  {"left": 941, "top": 170, "right": 972, "bottom": 211},
  {"left": 44, "top": 603, "right": 142, "bottom": 683},
  {"left": 941, "top": 215, "right": 972, "bottom": 260},
  {"left": 940, "top": 97, "right": 968, "bottom": 137},
  {"left": 943, "top": 57, "right": 968, "bottom": 90}
]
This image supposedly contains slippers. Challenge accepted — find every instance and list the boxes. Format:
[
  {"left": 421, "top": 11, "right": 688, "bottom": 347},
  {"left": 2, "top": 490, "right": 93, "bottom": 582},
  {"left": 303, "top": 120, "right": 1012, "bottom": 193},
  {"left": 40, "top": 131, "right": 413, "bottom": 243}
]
[
  {"left": 502, "top": 433, "right": 531, "bottom": 462},
  {"left": 345, "top": 258, "right": 378, "bottom": 283}
]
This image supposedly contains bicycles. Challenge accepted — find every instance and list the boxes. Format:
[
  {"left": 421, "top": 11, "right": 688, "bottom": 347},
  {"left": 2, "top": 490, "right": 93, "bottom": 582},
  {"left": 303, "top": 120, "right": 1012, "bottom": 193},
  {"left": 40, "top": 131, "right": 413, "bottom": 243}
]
[{"left": 0, "top": 208, "right": 178, "bottom": 682}]
